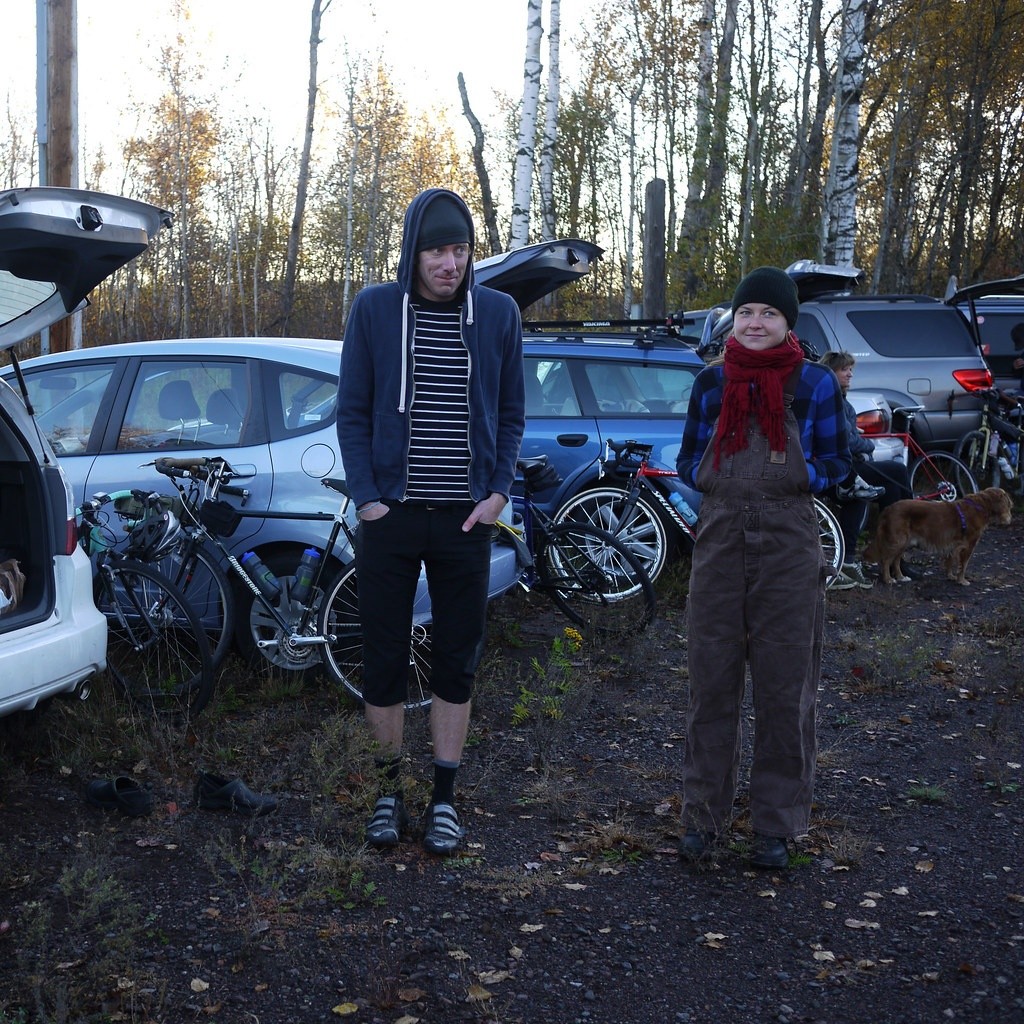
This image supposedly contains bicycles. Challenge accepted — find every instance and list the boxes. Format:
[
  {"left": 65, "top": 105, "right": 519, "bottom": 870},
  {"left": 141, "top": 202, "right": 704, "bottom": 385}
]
[
  {"left": 546, "top": 438, "right": 845, "bottom": 605},
  {"left": 76, "top": 489, "right": 215, "bottom": 723},
  {"left": 814, "top": 404, "right": 980, "bottom": 543},
  {"left": 947, "top": 387, "right": 1024, "bottom": 506},
  {"left": 510, "top": 453, "right": 659, "bottom": 639},
  {"left": 101, "top": 455, "right": 486, "bottom": 715}
]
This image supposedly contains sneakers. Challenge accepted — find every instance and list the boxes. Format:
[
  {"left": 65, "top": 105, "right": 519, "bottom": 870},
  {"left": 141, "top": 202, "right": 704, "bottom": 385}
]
[
  {"left": 422, "top": 801, "right": 462, "bottom": 856},
  {"left": 836, "top": 475, "right": 886, "bottom": 502},
  {"left": 828, "top": 575, "right": 857, "bottom": 590},
  {"left": 839, "top": 561, "right": 873, "bottom": 590},
  {"left": 367, "top": 796, "right": 409, "bottom": 847}
]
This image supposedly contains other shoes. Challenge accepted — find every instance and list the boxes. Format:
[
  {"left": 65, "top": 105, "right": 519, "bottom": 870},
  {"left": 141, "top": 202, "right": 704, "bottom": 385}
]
[
  {"left": 87, "top": 777, "right": 151, "bottom": 818},
  {"left": 900, "top": 558, "right": 923, "bottom": 577},
  {"left": 872, "top": 557, "right": 895, "bottom": 576},
  {"left": 195, "top": 774, "right": 279, "bottom": 818}
]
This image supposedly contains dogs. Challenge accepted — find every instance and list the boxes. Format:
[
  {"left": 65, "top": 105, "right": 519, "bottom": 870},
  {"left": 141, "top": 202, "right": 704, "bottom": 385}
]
[{"left": 862, "top": 488, "right": 1013, "bottom": 586}]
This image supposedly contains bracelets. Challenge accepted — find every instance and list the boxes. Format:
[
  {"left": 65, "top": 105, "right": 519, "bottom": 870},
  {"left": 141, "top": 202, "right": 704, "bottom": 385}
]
[{"left": 358, "top": 500, "right": 382, "bottom": 514}]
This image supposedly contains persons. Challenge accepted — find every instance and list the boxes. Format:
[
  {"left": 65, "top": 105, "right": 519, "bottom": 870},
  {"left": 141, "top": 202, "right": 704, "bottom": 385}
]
[
  {"left": 680, "top": 266, "right": 922, "bottom": 867},
  {"left": 335, "top": 190, "right": 527, "bottom": 850}
]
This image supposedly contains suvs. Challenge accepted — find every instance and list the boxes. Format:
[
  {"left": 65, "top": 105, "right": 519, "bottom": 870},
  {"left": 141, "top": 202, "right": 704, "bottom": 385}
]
[
  {"left": 0, "top": 184, "right": 178, "bottom": 732},
  {"left": 538, "top": 258, "right": 1024, "bottom": 509},
  {"left": 167, "top": 236, "right": 711, "bottom": 595},
  {"left": 0, "top": 338, "right": 531, "bottom": 686}
]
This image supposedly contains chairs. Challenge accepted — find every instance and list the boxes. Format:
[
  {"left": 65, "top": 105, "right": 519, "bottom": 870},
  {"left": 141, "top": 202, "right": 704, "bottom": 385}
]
[{"left": 158, "top": 379, "right": 243, "bottom": 448}]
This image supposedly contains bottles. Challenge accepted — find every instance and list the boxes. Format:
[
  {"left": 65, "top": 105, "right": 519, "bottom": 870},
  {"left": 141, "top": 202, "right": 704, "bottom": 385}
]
[
  {"left": 989, "top": 431, "right": 999, "bottom": 455},
  {"left": 291, "top": 547, "right": 320, "bottom": 602},
  {"left": 669, "top": 492, "right": 698, "bottom": 527},
  {"left": 1002, "top": 442, "right": 1012, "bottom": 457},
  {"left": 1011, "top": 443, "right": 1017, "bottom": 464},
  {"left": 513, "top": 512, "right": 527, "bottom": 543},
  {"left": 241, "top": 551, "right": 280, "bottom": 600},
  {"left": 998, "top": 457, "right": 1014, "bottom": 479}
]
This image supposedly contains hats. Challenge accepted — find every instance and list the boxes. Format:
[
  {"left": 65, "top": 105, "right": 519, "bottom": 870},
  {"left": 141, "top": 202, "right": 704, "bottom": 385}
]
[
  {"left": 732, "top": 266, "right": 798, "bottom": 330},
  {"left": 415, "top": 195, "right": 472, "bottom": 252},
  {"left": 1011, "top": 323, "right": 1024, "bottom": 351}
]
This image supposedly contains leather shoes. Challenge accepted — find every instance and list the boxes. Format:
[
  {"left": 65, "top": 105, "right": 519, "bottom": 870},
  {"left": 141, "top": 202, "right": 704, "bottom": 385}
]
[
  {"left": 751, "top": 834, "right": 790, "bottom": 869},
  {"left": 678, "top": 828, "right": 721, "bottom": 858}
]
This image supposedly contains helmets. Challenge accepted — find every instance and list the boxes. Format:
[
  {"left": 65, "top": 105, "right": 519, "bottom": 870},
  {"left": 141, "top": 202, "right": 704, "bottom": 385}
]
[
  {"left": 135, "top": 510, "right": 181, "bottom": 563},
  {"left": 799, "top": 339, "right": 821, "bottom": 362}
]
